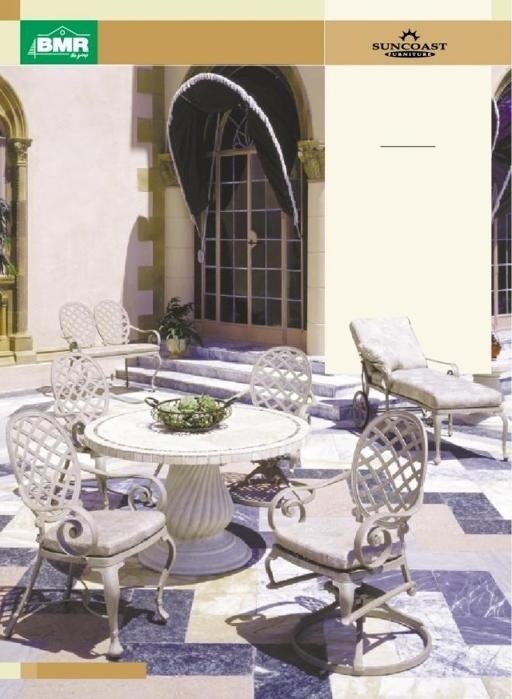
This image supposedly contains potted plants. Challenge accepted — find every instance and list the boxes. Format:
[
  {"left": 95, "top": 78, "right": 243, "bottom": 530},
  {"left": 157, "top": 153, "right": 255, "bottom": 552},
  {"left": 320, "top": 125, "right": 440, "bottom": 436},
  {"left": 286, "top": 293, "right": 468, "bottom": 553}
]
[{"left": 157, "top": 296, "right": 206, "bottom": 359}]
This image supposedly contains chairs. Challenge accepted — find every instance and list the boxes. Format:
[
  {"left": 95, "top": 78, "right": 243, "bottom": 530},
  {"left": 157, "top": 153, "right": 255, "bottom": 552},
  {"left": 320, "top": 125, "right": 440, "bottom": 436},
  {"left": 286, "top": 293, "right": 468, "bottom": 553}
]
[
  {"left": 3, "top": 409, "right": 179, "bottom": 661},
  {"left": 58, "top": 298, "right": 164, "bottom": 393},
  {"left": 229, "top": 345, "right": 320, "bottom": 509},
  {"left": 347, "top": 314, "right": 509, "bottom": 466},
  {"left": 263, "top": 407, "right": 436, "bottom": 678},
  {"left": 51, "top": 351, "right": 110, "bottom": 492}
]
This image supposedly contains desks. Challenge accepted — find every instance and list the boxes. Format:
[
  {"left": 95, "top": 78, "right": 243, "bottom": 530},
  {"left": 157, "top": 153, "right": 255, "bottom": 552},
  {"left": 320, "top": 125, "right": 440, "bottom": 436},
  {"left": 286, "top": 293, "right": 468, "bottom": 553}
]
[{"left": 81, "top": 404, "right": 312, "bottom": 577}]
